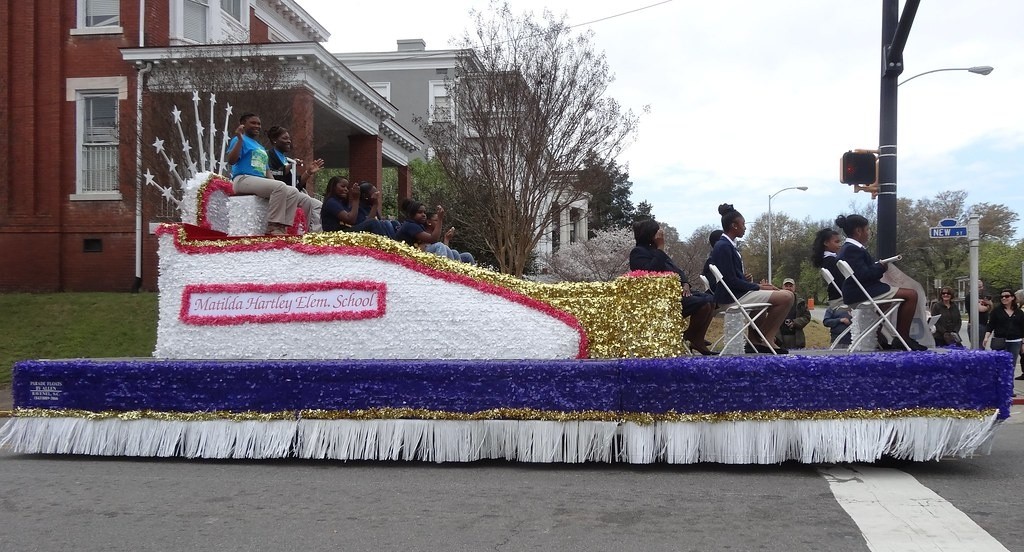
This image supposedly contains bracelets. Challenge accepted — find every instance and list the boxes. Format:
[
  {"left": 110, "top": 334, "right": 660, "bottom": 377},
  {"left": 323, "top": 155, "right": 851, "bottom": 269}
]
[
  {"left": 1022, "top": 343, "right": 1024, "bottom": 344},
  {"left": 305, "top": 170, "right": 312, "bottom": 177}
]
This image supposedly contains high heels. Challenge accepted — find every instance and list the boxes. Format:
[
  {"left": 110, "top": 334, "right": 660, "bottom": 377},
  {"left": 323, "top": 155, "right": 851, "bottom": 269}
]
[{"left": 682, "top": 330, "right": 720, "bottom": 355}]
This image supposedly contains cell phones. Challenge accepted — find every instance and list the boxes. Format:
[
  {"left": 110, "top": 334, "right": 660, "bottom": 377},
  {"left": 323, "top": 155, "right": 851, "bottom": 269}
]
[
  {"left": 437, "top": 205, "right": 444, "bottom": 211},
  {"left": 449, "top": 227, "right": 456, "bottom": 231}
]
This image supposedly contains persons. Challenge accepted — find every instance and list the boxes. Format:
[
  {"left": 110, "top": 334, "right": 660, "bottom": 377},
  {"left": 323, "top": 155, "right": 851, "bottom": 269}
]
[
  {"left": 226, "top": 111, "right": 300, "bottom": 235},
  {"left": 630, "top": 214, "right": 720, "bottom": 356},
  {"left": 927, "top": 286, "right": 962, "bottom": 347},
  {"left": 812, "top": 213, "right": 929, "bottom": 351},
  {"left": 982, "top": 289, "right": 1024, "bottom": 397},
  {"left": 965, "top": 279, "right": 993, "bottom": 348},
  {"left": 775, "top": 277, "right": 811, "bottom": 349},
  {"left": 320, "top": 174, "right": 476, "bottom": 266},
  {"left": 702, "top": 203, "right": 794, "bottom": 354},
  {"left": 268, "top": 126, "right": 324, "bottom": 233}
]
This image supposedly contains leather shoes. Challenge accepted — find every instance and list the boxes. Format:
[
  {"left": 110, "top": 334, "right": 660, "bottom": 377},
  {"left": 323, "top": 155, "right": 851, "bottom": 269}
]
[{"left": 744, "top": 335, "right": 788, "bottom": 354}]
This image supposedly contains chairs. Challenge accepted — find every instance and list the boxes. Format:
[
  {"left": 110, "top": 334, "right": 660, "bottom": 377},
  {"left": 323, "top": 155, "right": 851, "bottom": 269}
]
[
  {"left": 820, "top": 259, "right": 904, "bottom": 351},
  {"left": 699, "top": 263, "right": 773, "bottom": 355}
]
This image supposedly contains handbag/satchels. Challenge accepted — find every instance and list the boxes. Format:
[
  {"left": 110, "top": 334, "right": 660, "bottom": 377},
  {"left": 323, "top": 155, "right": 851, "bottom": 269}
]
[{"left": 990, "top": 338, "right": 1006, "bottom": 350}]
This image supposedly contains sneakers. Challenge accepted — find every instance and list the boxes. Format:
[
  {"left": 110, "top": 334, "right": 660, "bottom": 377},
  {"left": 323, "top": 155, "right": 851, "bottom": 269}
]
[{"left": 892, "top": 336, "right": 927, "bottom": 351}]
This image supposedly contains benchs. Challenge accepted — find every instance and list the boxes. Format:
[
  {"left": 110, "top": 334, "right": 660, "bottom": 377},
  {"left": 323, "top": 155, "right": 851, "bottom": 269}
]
[{"left": 226, "top": 191, "right": 270, "bottom": 239}]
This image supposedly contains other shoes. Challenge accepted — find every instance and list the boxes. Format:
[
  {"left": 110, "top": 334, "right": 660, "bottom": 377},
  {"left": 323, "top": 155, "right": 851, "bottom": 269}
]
[
  {"left": 1015, "top": 374, "right": 1024, "bottom": 380},
  {"left": 265, "top": 222, "right": 289, "bottom": 236}
]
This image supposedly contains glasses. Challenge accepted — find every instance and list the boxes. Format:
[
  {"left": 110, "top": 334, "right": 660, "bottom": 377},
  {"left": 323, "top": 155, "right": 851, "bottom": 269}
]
[
  {"left": 942, "top": 292, "right": 950, "bottom": 295},
  {"left": 999, "top": 295, "right": 1012, "bottom": 299}
]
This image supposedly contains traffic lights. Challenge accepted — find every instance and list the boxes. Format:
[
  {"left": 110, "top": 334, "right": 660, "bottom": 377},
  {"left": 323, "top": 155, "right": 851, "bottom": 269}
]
[{"left": 841, "top": 151, "right": 878, "bottom": 186}]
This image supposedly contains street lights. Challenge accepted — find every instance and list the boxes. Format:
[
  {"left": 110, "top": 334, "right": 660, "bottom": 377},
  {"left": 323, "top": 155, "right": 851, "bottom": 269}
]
[
  {"left": 767, "top": 186, "right": 809, "bottom": 285},
  {"left": 876, "top": 66, "right": 996, "bottom": 260}
]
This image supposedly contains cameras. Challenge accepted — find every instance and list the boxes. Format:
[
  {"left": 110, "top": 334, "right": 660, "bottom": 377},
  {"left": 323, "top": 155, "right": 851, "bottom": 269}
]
[{"left": 785, "top": 319, "right": 792, "bottom": 325}]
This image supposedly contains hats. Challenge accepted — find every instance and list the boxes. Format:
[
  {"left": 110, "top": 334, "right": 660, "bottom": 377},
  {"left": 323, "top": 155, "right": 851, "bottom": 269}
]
[
  {"left": 406, "top": 201, "right": 423, "bottom": 217},
  {"left": 633, "top": 220, "right": 660, "bottom": 243},
  {"left": 783, "top": 278, "right": 795, "bottom": 285}
]
